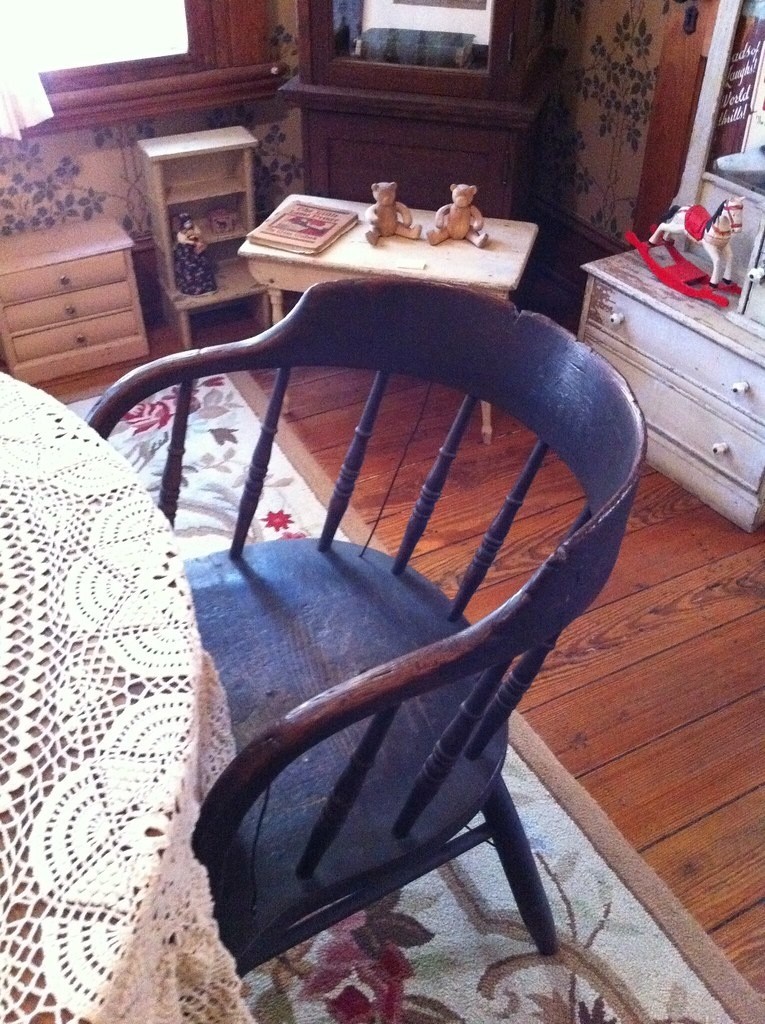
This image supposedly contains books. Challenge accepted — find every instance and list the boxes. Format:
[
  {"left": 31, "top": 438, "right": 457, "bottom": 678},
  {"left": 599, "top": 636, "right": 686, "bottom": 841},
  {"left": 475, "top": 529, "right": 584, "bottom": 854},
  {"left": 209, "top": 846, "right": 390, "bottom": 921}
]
[{"left": 246, "top": 199, "right": 359, "bottom": 254}]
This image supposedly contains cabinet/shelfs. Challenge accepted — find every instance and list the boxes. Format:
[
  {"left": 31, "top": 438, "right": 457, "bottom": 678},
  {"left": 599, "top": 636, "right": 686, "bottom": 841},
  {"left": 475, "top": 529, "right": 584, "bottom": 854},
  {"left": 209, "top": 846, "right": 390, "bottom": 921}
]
[
  {"left": 0, "top": 213, "right": 150, "bottom": 385},
  {"left": 278, "top": 0, "right": 545, "bottom": 219},
  {"left": 578, "top": 0, "right": 765, "bottom": 533},
  {"left": 138, "top": 126, "right": 271, "bottom": 350}
]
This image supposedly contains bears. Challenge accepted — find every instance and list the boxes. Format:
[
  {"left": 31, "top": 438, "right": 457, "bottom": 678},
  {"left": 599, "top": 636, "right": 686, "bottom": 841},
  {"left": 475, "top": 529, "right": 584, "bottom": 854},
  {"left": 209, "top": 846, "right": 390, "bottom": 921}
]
[
  {"left": 363, "top": 181, "right": 422, "bottom": 246},
  {"left": 426, "top": 183, "right": 489, "bottom": 249}
]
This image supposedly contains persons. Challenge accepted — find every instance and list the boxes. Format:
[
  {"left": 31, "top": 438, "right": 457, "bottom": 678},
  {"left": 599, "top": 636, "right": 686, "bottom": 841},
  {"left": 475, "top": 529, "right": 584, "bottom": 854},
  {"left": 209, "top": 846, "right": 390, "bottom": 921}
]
[{"left": 175, "top": 212, "right": 218, "bottom": 296}]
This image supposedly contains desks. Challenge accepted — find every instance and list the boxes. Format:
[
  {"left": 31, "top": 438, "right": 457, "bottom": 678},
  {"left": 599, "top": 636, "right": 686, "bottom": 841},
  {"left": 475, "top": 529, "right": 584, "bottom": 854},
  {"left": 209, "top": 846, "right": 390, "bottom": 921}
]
[
  {"left": 0, "top": 374, "right": 257, "bottom": 1024},
  {"left": 237, "top": 181, "right": 540, "bottom": 446}
]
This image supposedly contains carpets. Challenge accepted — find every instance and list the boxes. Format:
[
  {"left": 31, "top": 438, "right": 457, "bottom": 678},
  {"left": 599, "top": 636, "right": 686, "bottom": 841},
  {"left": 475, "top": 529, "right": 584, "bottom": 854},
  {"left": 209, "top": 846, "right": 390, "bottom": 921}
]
[{"left": 57, "top": 373, "right": 765, "bottom": 1024}]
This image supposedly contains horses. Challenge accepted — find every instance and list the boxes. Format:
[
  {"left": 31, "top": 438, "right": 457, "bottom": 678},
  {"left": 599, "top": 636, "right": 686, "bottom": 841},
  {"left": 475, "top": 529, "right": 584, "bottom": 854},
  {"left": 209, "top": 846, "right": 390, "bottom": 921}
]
[{"left": 648, "top": 195, "right": 747, "bottom": 289}]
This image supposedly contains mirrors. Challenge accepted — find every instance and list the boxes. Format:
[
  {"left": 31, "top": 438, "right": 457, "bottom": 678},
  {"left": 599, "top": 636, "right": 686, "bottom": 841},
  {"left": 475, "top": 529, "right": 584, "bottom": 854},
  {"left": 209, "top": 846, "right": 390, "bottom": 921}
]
[
  {"left": 333, "top": 0, "right": 492, "bottom": 72},
  {"left": 705, "top": 0, "right": 765, "bottom": 196}
]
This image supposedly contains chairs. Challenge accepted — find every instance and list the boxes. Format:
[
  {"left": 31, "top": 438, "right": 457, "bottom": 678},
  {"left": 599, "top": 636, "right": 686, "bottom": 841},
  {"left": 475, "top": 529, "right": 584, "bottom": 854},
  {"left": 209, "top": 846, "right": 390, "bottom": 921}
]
[{"left": 84, "top": 276, "right": 650, "bottom": 985}]
